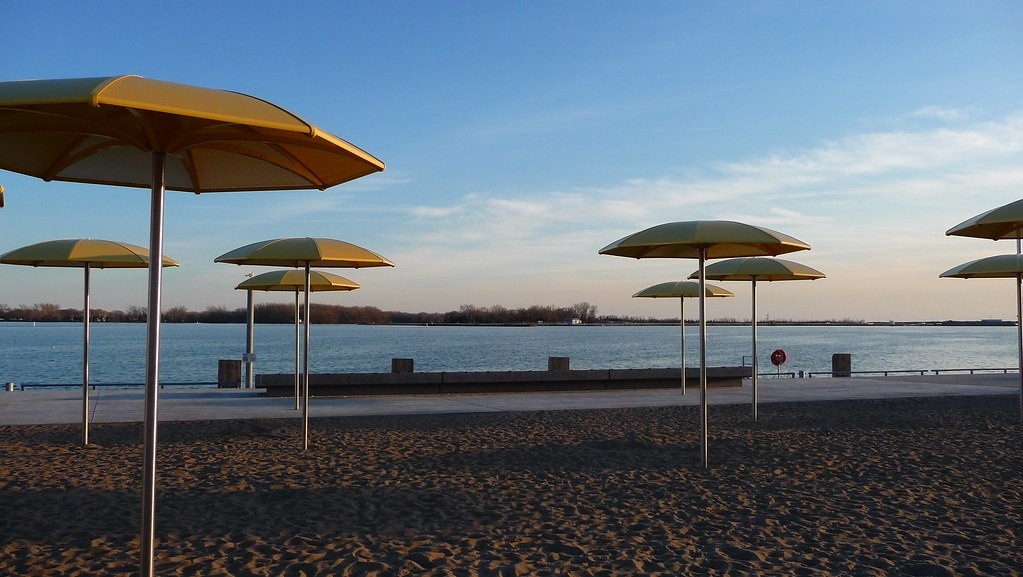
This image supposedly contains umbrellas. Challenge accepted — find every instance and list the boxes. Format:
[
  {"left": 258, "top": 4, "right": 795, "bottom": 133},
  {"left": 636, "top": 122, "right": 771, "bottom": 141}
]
[
  {"left": 0, "top": 76, "right": 395, "bottom": 577},
  {"left": 945, "top": 196, "right": 1023, "bottom": 256},
  {"left": 597, "top": 221, "right": 827, "bottom": 470},
  {"left": 939, "top": 255, "right": 1023, "bottom": 426}
]
